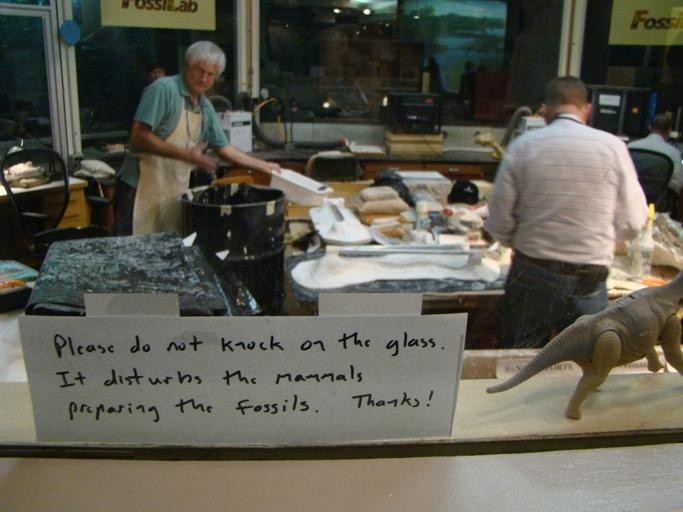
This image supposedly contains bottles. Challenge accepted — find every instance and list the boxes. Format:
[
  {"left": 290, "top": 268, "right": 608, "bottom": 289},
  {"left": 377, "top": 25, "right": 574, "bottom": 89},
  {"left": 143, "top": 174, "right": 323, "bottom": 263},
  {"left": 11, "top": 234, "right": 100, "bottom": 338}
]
[
  {"left": 630, "top": 217, "right": 655, "bottom": 279},
  {"left": 420, "top": 55, "right": 431, "bottom": 93}
]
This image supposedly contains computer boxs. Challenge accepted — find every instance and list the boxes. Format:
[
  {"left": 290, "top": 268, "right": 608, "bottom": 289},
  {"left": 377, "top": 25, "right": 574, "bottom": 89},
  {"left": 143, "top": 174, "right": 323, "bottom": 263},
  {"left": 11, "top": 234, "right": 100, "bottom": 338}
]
[{"left": 587, "top": 84, "right": 657, "bottom": 138}]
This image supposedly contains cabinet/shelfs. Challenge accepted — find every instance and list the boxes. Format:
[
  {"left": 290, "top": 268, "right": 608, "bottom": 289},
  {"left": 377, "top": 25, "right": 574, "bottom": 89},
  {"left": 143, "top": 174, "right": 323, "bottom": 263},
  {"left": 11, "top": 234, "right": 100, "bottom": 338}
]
[{"left": 225, "top": 158, "right": 482, "bottom": 185}]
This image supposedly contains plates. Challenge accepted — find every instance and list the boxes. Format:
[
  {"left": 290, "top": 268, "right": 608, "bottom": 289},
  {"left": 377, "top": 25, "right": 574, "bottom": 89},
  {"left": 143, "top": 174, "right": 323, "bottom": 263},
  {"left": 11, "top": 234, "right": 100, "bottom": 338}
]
[
  {"left": 308, "top": 204, "right": 372, "bottom": 244},
  {"left": 268, "top": 165, "right": 334, "bottom": 195}
]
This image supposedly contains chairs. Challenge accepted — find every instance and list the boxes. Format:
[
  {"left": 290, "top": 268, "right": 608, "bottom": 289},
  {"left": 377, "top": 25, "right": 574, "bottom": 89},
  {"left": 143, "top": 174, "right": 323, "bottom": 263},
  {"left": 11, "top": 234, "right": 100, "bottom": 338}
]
[
  {"left": 628, "top": 148, "right": 676, "bottom": 219},
  {"left": 0, "top": 148, "right": 112, "bottom": 261}
]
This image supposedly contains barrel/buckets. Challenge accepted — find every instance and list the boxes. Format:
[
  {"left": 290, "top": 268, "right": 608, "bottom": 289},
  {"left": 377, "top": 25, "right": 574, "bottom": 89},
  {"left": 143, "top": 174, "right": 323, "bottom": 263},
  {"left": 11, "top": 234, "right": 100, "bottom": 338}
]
[{"left": 179, "top": 183, "right": 285, "bottom": 316}]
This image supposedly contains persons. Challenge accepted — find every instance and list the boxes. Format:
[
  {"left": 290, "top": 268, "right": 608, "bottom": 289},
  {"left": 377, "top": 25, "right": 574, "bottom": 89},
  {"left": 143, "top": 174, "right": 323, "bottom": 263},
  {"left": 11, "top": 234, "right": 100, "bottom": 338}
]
[
  {"left": 482, "top": 76, "right": 650, "bottom": 350},
  {"left": 626, "top": 112, "right": 683, "bottom": 196},
  {"left": 111, "top": 38, "right": 281, "bottom": 236},
  {"left": 147, "top": 63, "right": 167, "bottom": 83}
]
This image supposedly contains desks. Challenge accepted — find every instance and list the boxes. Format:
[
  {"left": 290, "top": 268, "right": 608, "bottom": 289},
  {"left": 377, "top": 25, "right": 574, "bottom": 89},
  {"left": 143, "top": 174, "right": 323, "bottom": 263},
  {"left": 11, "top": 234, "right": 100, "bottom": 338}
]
[{"left": 0, "top": 173, "right": 89, "bottom": 233}]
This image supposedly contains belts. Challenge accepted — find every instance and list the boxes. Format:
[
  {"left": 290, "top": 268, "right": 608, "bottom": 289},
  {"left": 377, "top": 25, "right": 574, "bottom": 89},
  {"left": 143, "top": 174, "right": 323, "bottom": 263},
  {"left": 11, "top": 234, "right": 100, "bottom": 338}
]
[{"left": 530, "top": 257, "right": 607, "bottom": 278}]
